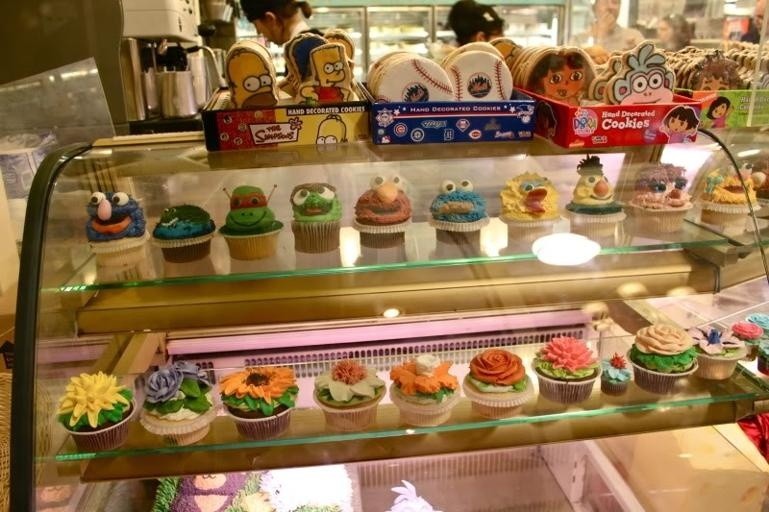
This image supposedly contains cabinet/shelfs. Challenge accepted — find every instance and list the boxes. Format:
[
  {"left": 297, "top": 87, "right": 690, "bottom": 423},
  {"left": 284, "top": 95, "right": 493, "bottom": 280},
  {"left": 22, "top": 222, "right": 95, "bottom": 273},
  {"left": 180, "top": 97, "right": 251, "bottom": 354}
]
[
  {"left": 4, "top": 127, "right": 769, "bottom": 511},
  {"left": 705, "top": 111, "right": 767, "bottom": 281}
]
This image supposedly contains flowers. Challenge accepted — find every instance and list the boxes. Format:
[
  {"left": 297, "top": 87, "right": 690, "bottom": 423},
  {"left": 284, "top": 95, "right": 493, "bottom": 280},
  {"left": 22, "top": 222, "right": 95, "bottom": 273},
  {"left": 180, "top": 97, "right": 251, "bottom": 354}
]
[
  {"left": 633, "top": 322, "right": 698, "bottom": 371},
  {"left": 391, "top": 354, "right": 461, "bottom": 399},
  {"left": 467, "top": 349, "right": 528, "bottom": 394},
  {"left": 217, "top": 367, "right": 301, "bottom": 412},
  {"left": 142, "top": 360, "right": 213, "bottom": 420},
  {"left": 56, "top": 371, "right": 132, "bottom": 427},
  {"left": 601, "top": 352, "right": 630, "bottom": 382},
  {"left": 533, "top": 334, "right": 601, "bottom": 381},
  {"left": 314, "top": 363, "right": 384, "bottom": 401}
]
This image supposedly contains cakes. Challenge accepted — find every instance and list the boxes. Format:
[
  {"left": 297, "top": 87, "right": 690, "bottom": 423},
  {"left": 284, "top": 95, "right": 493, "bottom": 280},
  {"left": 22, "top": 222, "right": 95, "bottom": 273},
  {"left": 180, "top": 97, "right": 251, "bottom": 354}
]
[
  {"left": 627, "top": 324, "right": 699, "bottom": 395},
  {"left": 463, "top": 350, "right": 533, "bottom": 419},
  {"left": 151, "top": 206, "right": 216, "bottom": 263},
  {"left": 390, "top": 354, "right": 459, "bottom": 426},
  {"left": 219, "top": 368, "right": 298, "bottom": 439},
  {"left": 313, "top": 361, "right": 386, "bottom": 430},
  {"left": 752, "top": 156, "right": 769, "bottom": 208},
  {"left": 732, "top": 323, "right": 763, "bottom": 362},
  {"left": 601, "top": 354, "right": 630, "bottom": 395},
  {"left": 746, "top": 314, "right": 769, "bottom": 340},
  {"left": 498, "top": 173, "right": 561, "bottom": 245},
  {"left": 628, "top": 164, "right": 693, "bottom": 234},
  {"left": 353, "top": 173, "right": 412, "bottom": 248},
  {"left": 56, "top": 371, "right": 137, "bottom": 451},
  {"left": 758, "top": 340, "right": 769, "bottom": 376},
  {"left": 531, "top": 335, "right": 600, "bottom": 404},
  {"left": 219, "top": 185, "right": 282, "bottom": 260},
  {"left": 86, "top": 191, "right": 150, "bottom": 268},
  {"left": 152, "top": 462, "right": 362, "bottom": 512},
  {"left": 140, "top": 361, "right": 216, "bottom": 446},
  {"left": 291, "top": 183, "right": 340, "bottom": 253},
  {"left": 565, "top": 154, "right": 626, "bottom": 239},
  {"left": 699, "top": 159, "right": 761, "bottom": 226},
  {"left": 391, "top": 479, "right": 434, "bottom": 511},
  {"left": 429, "top": 181, "right": 489, "bottom": 245}
]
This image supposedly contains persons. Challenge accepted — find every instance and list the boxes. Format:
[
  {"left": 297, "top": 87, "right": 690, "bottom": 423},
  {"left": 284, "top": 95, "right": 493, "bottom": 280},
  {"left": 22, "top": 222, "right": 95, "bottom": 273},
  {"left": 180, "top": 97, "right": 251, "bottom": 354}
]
[
  {"left": 741, "top": 18, "right": 759, "bottom": 44},
  {"left": 659, "top": 14, "right": 696, "bottom": 50},
  {"left": 427, "top": 0, "right": 507, "bottom": 63},
  {"left": 242, "top": 0, "right": 326, "bottom": 46},
  {"left": 571, "top": 0, "right": 644, "bottom": 56}
]
